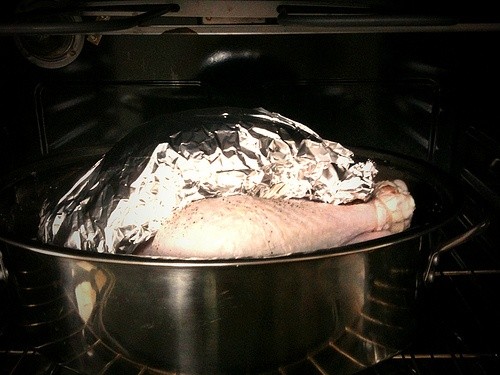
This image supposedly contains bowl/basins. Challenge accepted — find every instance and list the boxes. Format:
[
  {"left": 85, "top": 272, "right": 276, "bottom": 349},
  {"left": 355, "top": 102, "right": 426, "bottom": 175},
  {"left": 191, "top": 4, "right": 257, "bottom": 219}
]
[{"left": 0, "top": 149, "right": 455, "bottom": 371}]
